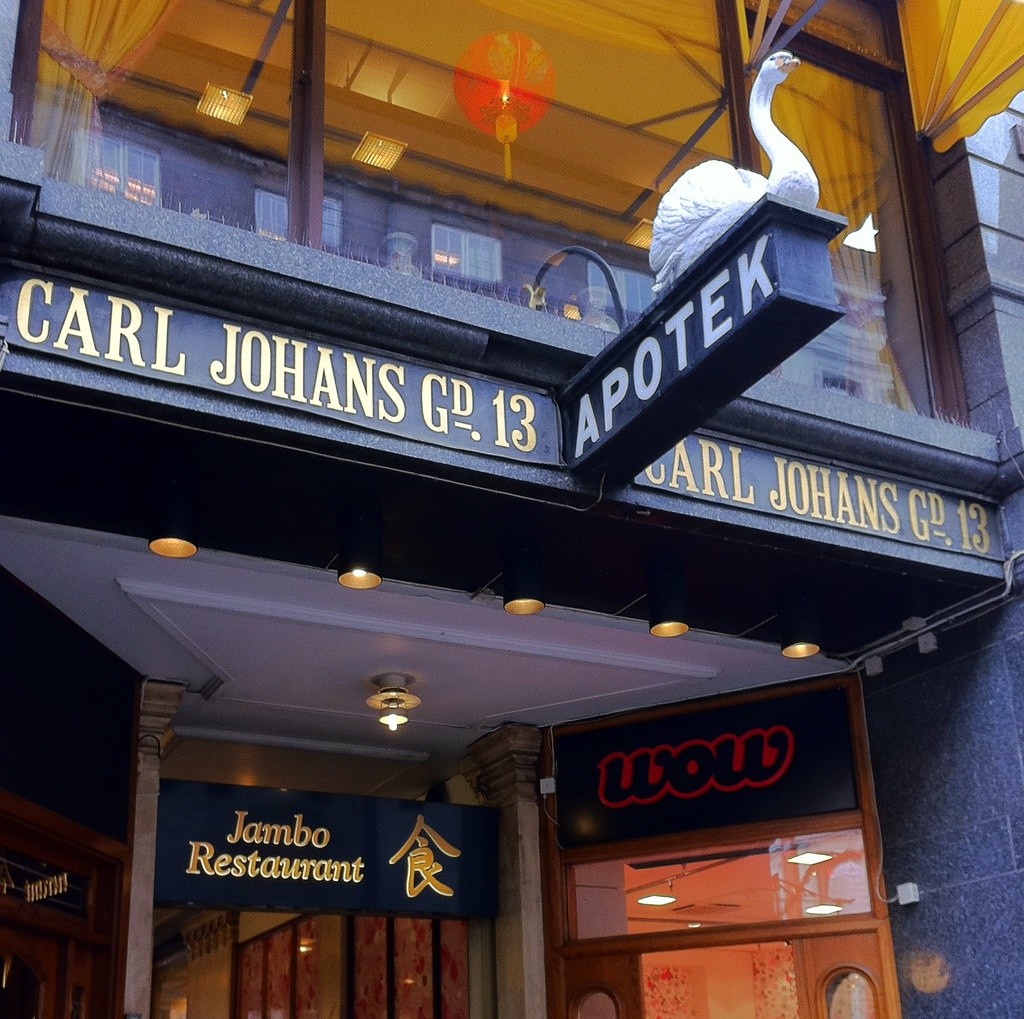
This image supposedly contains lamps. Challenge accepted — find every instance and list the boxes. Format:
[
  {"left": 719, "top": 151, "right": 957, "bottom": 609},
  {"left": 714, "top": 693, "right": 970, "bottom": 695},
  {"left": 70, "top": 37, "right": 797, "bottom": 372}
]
[
  {"left": 647, "top": 567, "right": 689, "bottom": 638},
  {"left": 337, "top": 498, "right": 382, "bottom": 591},
  {"left": 197, "top": 81, "right": 253, "bottom": 126},
  {"left": 365, "top": 673, "right": 422, "bottom": 731},
  {"left": 779, "top": 585, "right": 820, "bottom": 660},
  {"left": 499, "top": 536, "right": 546, "bottom": 615},
  {"left": 350, "top": 130, "right": 408, "bottom": 172},
  {"left": 624, "top": 218, "right": 653, "bottom": 250},
  {"left": 149, "top": 502, "right": 198, "bottom": 559}
]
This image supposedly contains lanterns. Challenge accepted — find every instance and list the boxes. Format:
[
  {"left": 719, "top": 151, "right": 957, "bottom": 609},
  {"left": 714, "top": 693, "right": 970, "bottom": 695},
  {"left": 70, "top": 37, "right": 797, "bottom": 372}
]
[{"left": 453, "top": 32, "right": 556, "bottom": 183}]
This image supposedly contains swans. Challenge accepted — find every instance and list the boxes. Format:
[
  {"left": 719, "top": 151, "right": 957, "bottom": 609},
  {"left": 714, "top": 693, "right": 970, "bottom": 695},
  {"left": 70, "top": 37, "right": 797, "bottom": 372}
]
[{"left": 648, "top": 50, "right": 821, "bottom": 298}]
